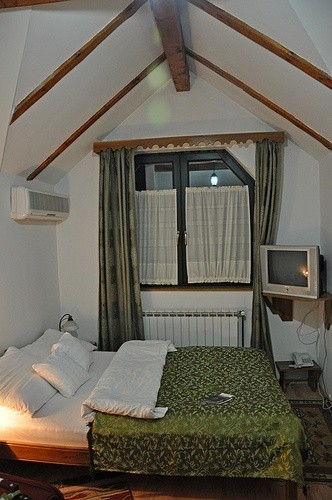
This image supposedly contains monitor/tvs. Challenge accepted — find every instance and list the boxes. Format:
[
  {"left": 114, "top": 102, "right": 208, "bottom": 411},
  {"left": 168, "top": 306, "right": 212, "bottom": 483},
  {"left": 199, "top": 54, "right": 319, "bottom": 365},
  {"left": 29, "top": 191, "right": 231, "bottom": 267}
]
[{"left": 259, "top": 245, "right": 323, "bottom": 299}]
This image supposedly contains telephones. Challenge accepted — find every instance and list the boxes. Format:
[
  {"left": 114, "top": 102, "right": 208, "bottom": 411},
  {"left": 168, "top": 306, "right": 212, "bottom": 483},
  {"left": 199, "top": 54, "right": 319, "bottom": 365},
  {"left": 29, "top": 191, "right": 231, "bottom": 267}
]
[{"left": 291, "top": 352, "right": 315, "bottom": 367}]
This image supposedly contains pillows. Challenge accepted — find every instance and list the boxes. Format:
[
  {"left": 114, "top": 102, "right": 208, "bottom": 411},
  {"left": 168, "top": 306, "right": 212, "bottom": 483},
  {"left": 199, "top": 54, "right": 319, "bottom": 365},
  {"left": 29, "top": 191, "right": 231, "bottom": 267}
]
[
  {"left": 52, "top": 332, "right": 97, "bottom": 371},
  {"left": 20, "top": 329, "right": 98, "bottom": 359},
  {"left": 0, "top": 346, "right": 57, "bottom": 418},
  {"left": 31, "top": 348, "right": 93, "bottom": 398}
]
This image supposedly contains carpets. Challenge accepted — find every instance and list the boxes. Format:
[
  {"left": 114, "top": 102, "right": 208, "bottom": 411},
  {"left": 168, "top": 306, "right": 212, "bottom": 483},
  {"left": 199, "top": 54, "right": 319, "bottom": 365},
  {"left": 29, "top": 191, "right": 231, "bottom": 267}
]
[{"left": 288, "top": 399, "right": 332, "bottom": 483}]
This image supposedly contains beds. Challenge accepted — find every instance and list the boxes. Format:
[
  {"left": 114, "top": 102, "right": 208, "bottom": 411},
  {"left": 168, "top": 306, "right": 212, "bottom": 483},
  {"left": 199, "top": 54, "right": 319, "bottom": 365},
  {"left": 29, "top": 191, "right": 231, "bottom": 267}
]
[{"left": 0, "top": 340, "right": 313, "bottom": 500}]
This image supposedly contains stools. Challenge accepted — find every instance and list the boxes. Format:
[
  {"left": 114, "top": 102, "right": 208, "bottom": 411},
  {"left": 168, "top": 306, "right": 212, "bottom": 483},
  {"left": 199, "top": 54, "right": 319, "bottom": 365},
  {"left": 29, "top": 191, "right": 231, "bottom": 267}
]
[{"left": 275, "top": 360, "right": 323, "bottom": 392}]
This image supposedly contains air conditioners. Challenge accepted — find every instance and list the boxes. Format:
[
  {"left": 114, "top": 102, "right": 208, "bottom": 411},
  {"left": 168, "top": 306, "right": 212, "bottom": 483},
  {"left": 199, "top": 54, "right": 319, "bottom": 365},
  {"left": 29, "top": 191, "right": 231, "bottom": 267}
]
[{"left": 10, "top": 186, "right": 70, "bottom": 221}]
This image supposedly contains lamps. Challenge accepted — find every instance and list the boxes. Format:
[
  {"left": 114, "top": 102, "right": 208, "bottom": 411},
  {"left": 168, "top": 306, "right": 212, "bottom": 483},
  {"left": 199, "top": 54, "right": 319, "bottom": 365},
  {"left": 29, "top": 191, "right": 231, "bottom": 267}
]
[
  {"left": 210, "top": 161, "right": 218, "bottom": 186},
  {"left": 59, "top": 314, "right": 80, "bottom": 332}
]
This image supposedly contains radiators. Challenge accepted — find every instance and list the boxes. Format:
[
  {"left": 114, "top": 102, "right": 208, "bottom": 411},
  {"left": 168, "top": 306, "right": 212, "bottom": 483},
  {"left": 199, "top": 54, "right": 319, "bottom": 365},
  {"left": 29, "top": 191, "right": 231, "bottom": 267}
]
[{"left": 142, "top": 309, "right": 246, "bottom": 347}]
